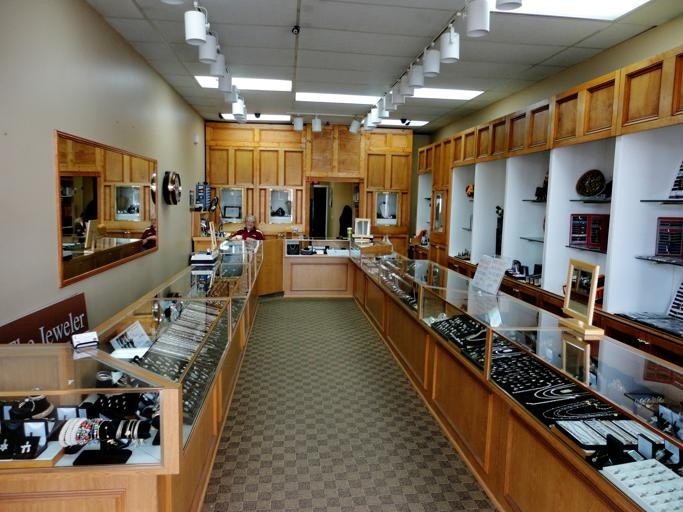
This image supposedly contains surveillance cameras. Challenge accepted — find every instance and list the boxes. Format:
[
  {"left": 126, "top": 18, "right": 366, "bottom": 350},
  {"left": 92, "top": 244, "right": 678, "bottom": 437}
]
[{"left": 254, "top": 112, "right": 260, "bottom": 118}]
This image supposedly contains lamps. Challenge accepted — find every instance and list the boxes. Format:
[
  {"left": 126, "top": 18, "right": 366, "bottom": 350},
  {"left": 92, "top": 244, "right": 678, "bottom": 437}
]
[
  {"left": 177, "top": 0, "right": 250, "bottom": 130},
  {"left": 363, "top": 2, "right": 487, "bottom": 136},
  {"left": 290, "top": 112, "right": 365, "bottom": 134}
]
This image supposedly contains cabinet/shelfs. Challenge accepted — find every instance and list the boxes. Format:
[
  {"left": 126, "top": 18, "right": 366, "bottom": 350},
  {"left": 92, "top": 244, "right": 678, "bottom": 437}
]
[{"left": 415, "top": 46, "right": 681, "bottom": 363}]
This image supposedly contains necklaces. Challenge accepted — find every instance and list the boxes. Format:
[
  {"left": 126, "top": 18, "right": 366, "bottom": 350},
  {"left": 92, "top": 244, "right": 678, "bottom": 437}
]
[
  {"left": 431, "top": 312, "right": 672, "bottom": 446},
  {"left": 58, "top": 270, "right": 244, "bottom": 420}
]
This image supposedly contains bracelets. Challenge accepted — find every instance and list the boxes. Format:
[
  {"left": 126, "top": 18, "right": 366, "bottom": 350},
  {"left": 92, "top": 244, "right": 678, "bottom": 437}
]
[
  {"left": 57, "top": 417, "right": 144, "bottom": 448},
  {"left": 378, "top": 272, "right": 418, "bottom": 306}
]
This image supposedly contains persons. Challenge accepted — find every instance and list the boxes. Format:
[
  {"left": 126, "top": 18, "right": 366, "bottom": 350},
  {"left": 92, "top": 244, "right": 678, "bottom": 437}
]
[
  {"left": 141, "top": 214, "right": 157, "bottom": 244},
  {"left": 71, "top": 211, "right": 89, "bottom": 243},
  {"left": 231, "top": 215, "right": 264, "bottom": 240}
]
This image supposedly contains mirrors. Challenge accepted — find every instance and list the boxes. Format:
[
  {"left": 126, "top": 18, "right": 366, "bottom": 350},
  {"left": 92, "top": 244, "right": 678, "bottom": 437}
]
[{"left": 54, "top": 130, "right": 161, "bottom": 291}]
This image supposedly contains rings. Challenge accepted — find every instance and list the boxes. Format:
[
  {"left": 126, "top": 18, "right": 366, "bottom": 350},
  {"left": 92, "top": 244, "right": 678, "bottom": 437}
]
[{"left": 611, "top": 463, "right": 683, "bottom": 512}]
